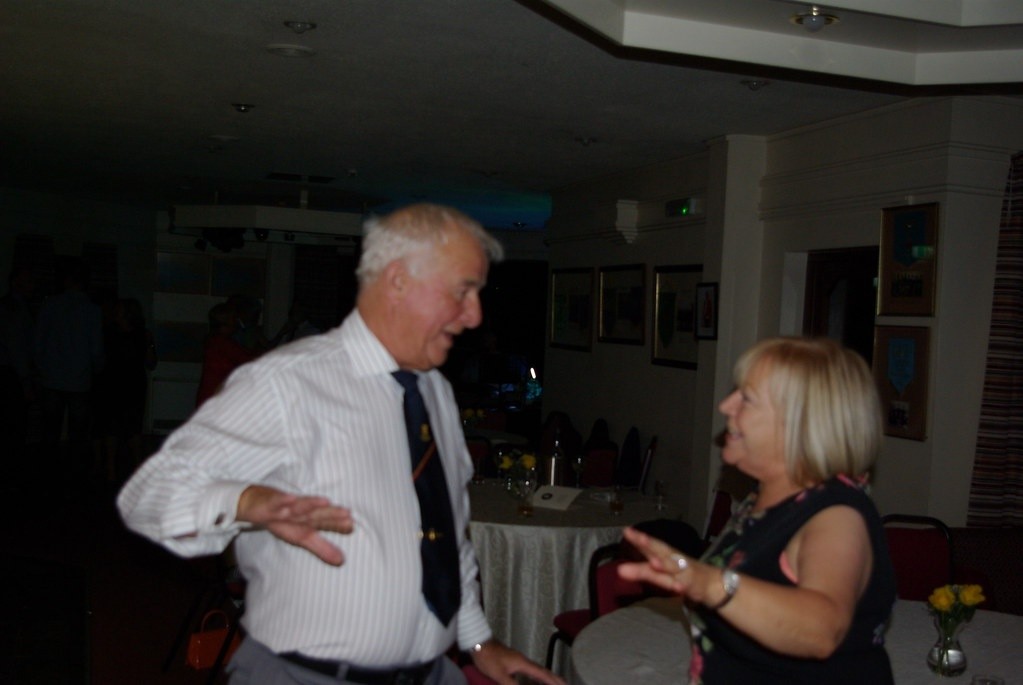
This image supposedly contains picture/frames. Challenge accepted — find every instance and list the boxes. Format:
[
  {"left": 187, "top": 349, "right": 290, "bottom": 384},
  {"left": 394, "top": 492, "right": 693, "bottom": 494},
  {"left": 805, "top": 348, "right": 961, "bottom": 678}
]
[
  {"left": 874, "top": 200, "right": 939, "bottom": 314},
  {"left": 873, "top": 324, "right": 930, "bottom": 441},
  {"left": 547, "top": 266, "right": 593, "bottom": 353},
  {"left": 696, "top": 281, "right": 720, "bottom": 341},
  {"left": 597, "top": 263, "right": 647, "bottom": 346},
  {"left": 653, "top": 263, "right": 704, "bottom": 371}
]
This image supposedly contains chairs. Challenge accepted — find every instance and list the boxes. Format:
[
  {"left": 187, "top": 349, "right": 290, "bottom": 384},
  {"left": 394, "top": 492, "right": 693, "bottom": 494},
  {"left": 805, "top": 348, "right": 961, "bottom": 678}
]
[{"left": 152, "top": 393, "right": 956, "bottom": 684}]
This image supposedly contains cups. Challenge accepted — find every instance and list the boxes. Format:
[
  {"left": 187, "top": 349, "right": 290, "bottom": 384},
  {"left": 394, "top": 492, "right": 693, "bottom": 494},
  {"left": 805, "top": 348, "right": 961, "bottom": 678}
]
[
  {"left": 609, "top": 486, "right": 625, "bottom": 516},
  {"left": 971, "top": 674, "right": 1005, "bottom": 685},
  {"left": 654, "top": 493, "right": 665, "bottom": 514},
  {"left": 471, "top": 445, "right": 540, "bottom": 520}
]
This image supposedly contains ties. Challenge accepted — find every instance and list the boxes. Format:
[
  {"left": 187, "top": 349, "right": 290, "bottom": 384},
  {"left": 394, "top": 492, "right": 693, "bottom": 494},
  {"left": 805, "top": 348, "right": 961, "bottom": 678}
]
[{"left": 391, "top": 368, "right": 463, "bottom": 628}]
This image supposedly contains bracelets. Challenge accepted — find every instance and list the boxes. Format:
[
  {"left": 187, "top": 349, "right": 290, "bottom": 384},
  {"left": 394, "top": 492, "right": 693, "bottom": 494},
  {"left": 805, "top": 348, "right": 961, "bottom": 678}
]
[{"left": 471, "top": 639, "right": 494, "bottom": 653}]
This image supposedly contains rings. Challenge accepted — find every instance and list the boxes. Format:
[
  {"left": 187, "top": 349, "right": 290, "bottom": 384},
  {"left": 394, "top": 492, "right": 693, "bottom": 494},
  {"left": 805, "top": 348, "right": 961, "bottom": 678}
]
[{"left": 674, "top": 553, "right": 687, "bottom": 569}]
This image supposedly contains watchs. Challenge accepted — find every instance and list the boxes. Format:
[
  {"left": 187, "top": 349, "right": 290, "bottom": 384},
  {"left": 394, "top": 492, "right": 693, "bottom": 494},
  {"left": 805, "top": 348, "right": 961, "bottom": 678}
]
[{"left": 710, "top": 568, "right": 739, "bottom": 611}]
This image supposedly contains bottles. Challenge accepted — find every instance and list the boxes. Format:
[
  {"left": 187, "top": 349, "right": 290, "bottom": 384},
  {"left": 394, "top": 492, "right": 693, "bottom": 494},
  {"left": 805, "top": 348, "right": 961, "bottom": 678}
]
[{"left": 542, "top": 439, "right": 565, "bottom": 488}]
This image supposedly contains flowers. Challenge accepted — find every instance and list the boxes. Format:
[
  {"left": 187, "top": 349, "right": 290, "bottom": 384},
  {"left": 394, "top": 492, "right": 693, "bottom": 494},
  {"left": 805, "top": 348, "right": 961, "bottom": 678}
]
[
  {"left": 515, "top": 452, "right": 536, "bottom": 480},
  {"left": 927, "top": 584, "right": 986, "bottom": 675},
  {"left": 497, "top": 454, "right": 517, "bottom": 472}
]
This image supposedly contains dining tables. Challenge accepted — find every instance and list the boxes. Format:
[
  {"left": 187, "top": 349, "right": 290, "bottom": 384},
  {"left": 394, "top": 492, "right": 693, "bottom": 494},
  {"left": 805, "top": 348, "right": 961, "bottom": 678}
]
[
  {"left": 461, "top": 469, "right": 680, "bottom": 670},
  {"left": 461, "top": 426, "right": 532, "bottom": 464},
  {"left": 566, "top": 595, "right": 1023, "bottom": 683}
]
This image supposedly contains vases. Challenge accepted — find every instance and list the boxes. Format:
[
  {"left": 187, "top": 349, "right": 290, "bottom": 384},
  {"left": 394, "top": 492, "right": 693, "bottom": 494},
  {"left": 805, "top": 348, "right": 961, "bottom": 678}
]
[
  {"left": 500, "top": 468, "right": 520, "bottom": 496},
  {"left": 519, "top": 468, "right": 538, "bottom": 514},
  {"left": 926, "top": 613, "right": 966, "bottom": 674}
]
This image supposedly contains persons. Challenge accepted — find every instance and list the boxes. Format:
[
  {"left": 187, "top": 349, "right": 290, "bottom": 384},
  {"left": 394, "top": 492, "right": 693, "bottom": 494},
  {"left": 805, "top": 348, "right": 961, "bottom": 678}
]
[
  {"left": 616, "top": 336, "right": 897, "bottom": 685},
  {"left": 116, "top": 205, "right": 566, "bottom": 685},
  {"left": 0, "top": 260, "right": 325, "bottom": 487}
]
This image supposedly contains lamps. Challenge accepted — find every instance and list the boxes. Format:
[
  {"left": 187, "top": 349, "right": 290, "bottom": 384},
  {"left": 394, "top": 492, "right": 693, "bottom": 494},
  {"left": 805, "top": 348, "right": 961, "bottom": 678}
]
[{"left": 789, "top": 4, "right": 839, "bottom": 35}]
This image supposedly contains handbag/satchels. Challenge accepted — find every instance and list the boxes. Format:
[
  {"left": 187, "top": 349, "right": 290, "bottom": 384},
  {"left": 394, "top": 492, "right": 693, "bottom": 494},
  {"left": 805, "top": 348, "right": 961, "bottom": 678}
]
[{"left": 186, "top": 610, "right": 240, "bottom": 669}]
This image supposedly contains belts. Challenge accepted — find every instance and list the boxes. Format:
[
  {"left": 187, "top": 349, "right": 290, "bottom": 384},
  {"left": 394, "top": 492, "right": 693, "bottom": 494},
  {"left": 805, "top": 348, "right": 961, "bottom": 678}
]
[{"left": 277, "top": 652, "right": 435, "bottom": 685}]
